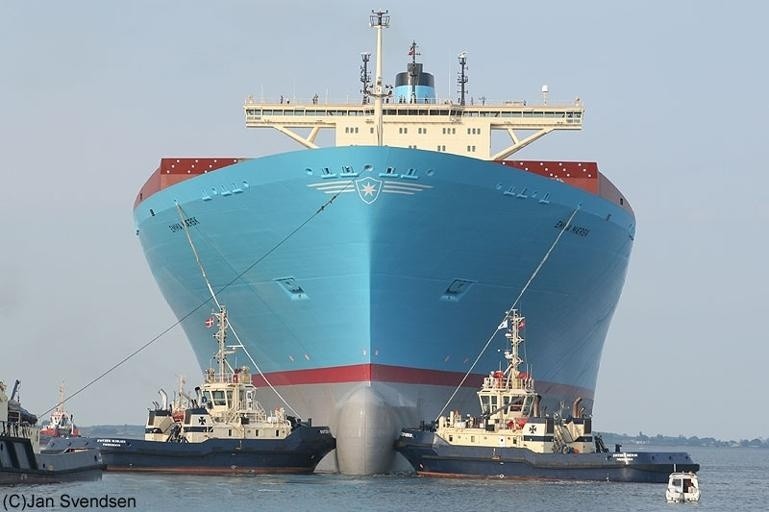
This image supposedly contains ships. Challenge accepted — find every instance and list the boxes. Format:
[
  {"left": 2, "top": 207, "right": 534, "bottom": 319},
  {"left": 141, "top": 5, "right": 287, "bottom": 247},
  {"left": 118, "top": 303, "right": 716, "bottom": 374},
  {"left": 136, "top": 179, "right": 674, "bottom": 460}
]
[{"left": 132, "top": 8, "right": 637, "bottom": 476}]
